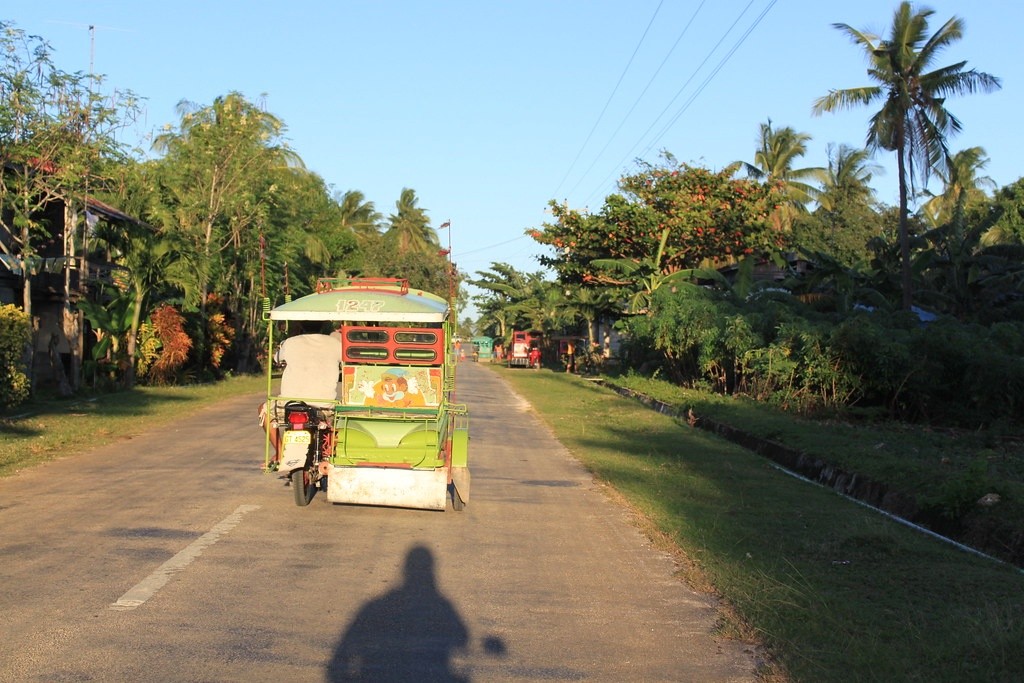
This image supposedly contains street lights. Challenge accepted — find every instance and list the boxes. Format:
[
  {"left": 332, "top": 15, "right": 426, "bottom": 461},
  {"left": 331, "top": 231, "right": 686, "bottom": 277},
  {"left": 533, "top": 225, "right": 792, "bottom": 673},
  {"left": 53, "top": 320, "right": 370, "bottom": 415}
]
[{"left": 437, "top": 219, "right": 453, "bottom": 324}]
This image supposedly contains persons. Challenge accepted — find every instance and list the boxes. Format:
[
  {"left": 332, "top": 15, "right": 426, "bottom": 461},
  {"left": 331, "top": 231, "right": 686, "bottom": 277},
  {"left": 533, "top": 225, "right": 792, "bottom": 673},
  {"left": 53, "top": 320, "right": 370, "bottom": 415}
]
[
  {"left": 495, "top": 342, "right": 502, "bottom": 362},
  {"left": 454, "top": 340, "right": 460, "bottom": 356},
  {"left": 258, "top": 321, "right": 342, "bottom": 469},
  {"left": 460, "top": 349, "right": 465, "bottom": 360},
  {"left": 472, "top": 342, "right": 478, "bottom": 354},
  {"left": 330, "top": 325, "right": 341, "bottom": 342}
]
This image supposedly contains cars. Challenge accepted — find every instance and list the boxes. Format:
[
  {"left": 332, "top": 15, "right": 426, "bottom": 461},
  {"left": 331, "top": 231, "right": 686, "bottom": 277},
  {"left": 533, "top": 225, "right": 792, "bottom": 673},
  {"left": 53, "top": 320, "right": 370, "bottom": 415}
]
[{"left": 451, "top": 335, "right": 462, "bottom": 344}]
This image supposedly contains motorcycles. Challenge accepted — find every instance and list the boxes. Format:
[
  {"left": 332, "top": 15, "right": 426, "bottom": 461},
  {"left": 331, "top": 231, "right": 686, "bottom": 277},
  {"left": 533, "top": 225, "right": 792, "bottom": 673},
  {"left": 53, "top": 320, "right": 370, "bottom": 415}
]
[
  {"left": 505, "top": 331, "right": 541, "bottom": 369},
  {"left": 470, "top": 335, "right": 496, "bottom": 362},
  {"left": 259, "top": 275, "right": 474, "bottom": 511}
]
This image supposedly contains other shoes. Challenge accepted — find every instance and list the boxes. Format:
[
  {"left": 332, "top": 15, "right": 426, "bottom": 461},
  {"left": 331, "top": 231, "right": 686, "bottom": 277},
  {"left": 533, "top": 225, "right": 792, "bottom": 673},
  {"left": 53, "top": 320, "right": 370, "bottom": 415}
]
[{"left": 260, "top": 460, "right": 279, "bottom": 471}]
